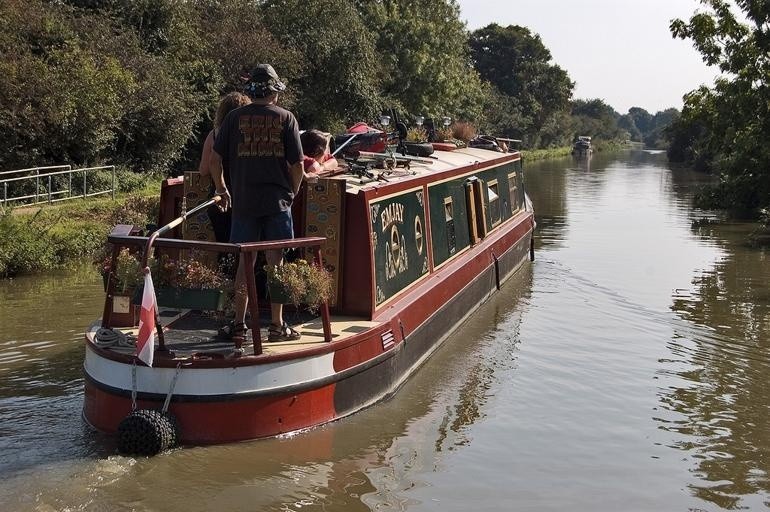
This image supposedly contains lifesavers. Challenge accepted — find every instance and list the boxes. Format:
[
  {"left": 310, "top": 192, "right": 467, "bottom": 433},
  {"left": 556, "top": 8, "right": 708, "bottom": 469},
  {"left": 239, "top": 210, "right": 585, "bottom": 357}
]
[{"left": 429, "top": 143, "right": 457, "bottom": 150}]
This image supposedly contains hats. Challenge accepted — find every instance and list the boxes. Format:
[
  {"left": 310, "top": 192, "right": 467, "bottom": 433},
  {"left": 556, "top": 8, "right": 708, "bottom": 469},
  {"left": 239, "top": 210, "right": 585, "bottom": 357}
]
[{"left": 243, "top": 63, "right": 286, "bottom": 99}]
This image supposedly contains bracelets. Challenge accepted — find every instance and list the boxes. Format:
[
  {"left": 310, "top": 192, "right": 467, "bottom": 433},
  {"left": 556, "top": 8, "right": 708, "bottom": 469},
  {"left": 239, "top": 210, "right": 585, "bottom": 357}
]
[
  {"left": 214, "top": 188, "right": 228, "bottom": 195},
  {"left": 320, "top": 163, "right": 326, "bottom": 170}
]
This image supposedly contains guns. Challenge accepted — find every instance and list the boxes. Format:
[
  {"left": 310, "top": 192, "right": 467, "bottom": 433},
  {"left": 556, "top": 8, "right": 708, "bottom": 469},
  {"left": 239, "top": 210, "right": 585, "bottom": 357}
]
[{"left": 344, "top": 157, "right": 417, "bottom": 184}]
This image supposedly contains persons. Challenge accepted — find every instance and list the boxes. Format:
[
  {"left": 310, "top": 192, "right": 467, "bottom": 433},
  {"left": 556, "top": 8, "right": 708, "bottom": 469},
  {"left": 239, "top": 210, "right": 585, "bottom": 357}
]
[
  {"left": 205, "top": 63, "right": 305, "bottom": 342},
  {"left": 299, "top": 129, "right": 339, "bottom": 183},
  {"left": 198, "top": 92, "right": 321, "bottom": 319}
]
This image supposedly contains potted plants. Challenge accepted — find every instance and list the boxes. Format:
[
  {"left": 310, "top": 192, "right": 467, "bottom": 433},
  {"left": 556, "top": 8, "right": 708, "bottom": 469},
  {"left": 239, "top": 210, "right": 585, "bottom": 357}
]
[
  {"left": 92, "top": 248, "right": 233, "bottom": 311},
  {"left": 267, "top": 260, "right": 337, "bottom": 315}
]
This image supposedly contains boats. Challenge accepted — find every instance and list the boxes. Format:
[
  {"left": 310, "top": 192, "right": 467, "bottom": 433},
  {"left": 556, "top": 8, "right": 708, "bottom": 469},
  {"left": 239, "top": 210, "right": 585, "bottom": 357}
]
[
  {"left": 77, "top": 112, "right": 539, "bottom": 454},
  {"left": 572, "top": 135, "right": 593, "bottom": 156}
]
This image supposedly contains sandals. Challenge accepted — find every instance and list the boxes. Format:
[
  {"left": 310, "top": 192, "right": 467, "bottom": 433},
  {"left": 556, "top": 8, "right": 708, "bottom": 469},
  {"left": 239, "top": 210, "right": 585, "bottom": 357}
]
[
  {"left": 268, "top": 322, "right": 301, "bottom": 343},
  {"left": 217, "top": 319, "right": 249, "bottom": 342}
]
